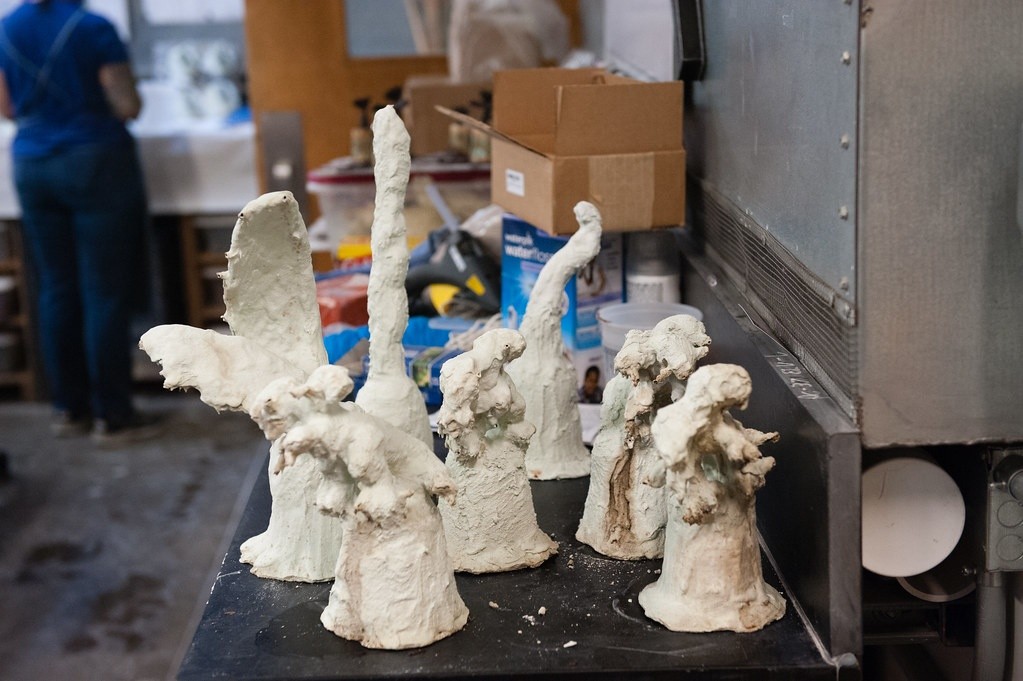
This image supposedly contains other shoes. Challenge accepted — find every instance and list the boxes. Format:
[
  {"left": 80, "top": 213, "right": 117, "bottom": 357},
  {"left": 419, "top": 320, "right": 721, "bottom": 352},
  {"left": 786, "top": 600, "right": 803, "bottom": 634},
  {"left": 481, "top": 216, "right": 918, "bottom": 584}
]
[
  {"left": 90, "top": 408, "right": 168, "bottom": 442},
  {"left": 48, "top": 407, "right": 88, "bottom": 433}
]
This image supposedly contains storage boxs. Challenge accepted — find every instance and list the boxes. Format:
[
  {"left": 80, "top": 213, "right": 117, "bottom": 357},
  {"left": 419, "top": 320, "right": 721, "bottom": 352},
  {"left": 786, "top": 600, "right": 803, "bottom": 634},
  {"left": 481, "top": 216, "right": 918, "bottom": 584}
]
[
  {"left": 315, "top": 272, "right": 368, "bottom": 328},
  {"left": 433, "top": 66, "right": 688, "bottom": 237},
  {"left": 362, "top": 343, "right": 465, "bottom": 409},
  {"left": 498, "top": 213, "right": 625, "bottom": 406}
]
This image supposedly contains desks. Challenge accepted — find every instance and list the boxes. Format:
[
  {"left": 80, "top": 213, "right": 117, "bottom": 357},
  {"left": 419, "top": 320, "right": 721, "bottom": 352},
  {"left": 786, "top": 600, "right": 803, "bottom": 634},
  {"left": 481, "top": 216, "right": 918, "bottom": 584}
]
[{"left": 0, "top": 81, "right": 259, "bottom": 216}]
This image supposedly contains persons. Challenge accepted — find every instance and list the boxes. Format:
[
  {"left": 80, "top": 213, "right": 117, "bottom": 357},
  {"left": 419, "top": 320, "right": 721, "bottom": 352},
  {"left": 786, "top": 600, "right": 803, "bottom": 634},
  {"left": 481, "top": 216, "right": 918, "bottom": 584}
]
[
  {"left": 0, "top": 0, "right": 179, "bottom": 446},
  {"left": 579, "top": 366, "right": 603, "bottom": 403}
]
